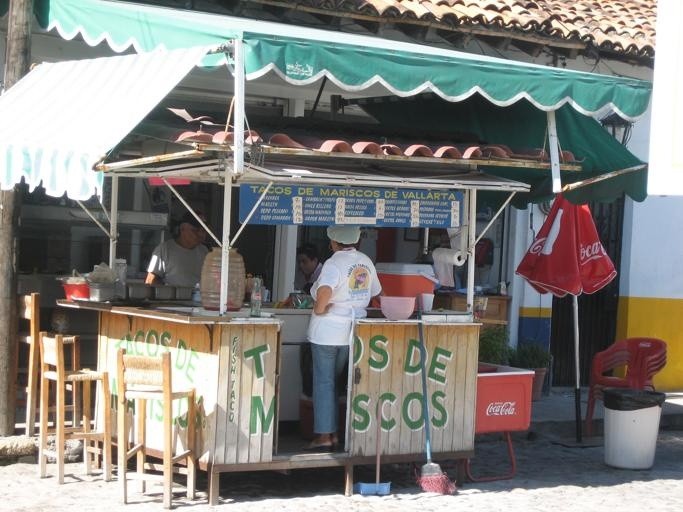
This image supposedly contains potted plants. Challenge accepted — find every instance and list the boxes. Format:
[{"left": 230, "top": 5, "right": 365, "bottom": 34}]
[{"left": 508, "top": 339, "right": 551, "bottom": 401}]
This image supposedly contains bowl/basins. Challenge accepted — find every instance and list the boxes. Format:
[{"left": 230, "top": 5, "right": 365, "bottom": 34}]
[{"left": 381, "top": 296, "right": 416, "bottom": 320}]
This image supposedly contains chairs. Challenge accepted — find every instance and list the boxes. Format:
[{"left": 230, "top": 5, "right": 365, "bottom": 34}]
[
  {"left": 115, "top": 347, "right": 200, "bottom": 507},
  {"left": 38, "top": 330, "right": 115, "bottom": 484},
  {"left": 14, "top": 291, "right": 78, "bottom": 436},
  {"left": 585, "top": 336, "right": 669, "bottom": 431}
]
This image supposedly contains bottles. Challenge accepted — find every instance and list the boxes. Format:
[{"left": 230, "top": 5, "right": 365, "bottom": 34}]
[
  {"left": 191, "top": 283, "right": 201, "bottom": 318},
  {"left": 473, "top": 286, "right": 483, "bottom": 322},
  {"left": 250, "top": 279, "right": 262, "bottom": 317}
]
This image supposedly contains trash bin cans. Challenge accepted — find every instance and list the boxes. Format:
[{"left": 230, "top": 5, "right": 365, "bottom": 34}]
[{"left": 603, "top": 389, "right": 665, "bottom": 469}]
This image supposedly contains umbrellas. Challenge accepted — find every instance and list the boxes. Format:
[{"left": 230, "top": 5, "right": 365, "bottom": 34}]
[{"left": 515, "top": 192, "right": 619, "bottom": 441}]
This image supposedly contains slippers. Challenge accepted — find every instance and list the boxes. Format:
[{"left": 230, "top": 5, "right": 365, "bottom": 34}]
[{"left": 302, "top": 438, "right": 339, "bottom": 451}]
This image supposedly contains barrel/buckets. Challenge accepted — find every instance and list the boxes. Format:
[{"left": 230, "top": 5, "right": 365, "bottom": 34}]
[
  {"left": 201, "top": 246, "right": 247, "bottom": 312},
  {"left": 62, "top": 284, "right": 90, "bottom": 302}
]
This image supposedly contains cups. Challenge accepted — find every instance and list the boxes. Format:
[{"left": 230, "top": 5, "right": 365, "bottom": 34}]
[{"left": 421, "top": 293, "right": 434, "bottom": 312}]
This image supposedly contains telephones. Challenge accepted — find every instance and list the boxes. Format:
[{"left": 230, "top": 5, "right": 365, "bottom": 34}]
[{"left": 474, "top": 237, "right": 493, "bottom": 267}]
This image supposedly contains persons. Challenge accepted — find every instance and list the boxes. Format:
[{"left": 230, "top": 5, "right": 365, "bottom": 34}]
[
  {"left": 144, "top": 209, "right": 210, "bottom": 288},
  {"left": 295, "top": 243, "right": 323, "bottom": 293},
  {"left": 302, "top": 225, "right": 383, "bottom": 452}
]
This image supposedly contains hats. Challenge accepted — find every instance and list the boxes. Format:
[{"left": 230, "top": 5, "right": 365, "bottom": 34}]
[
  {"left": 327, "top": 223, "right": 361, "bottom": 244},
  {"left": 180, "top": 210, "right": 210, "bottom": 228}
]
